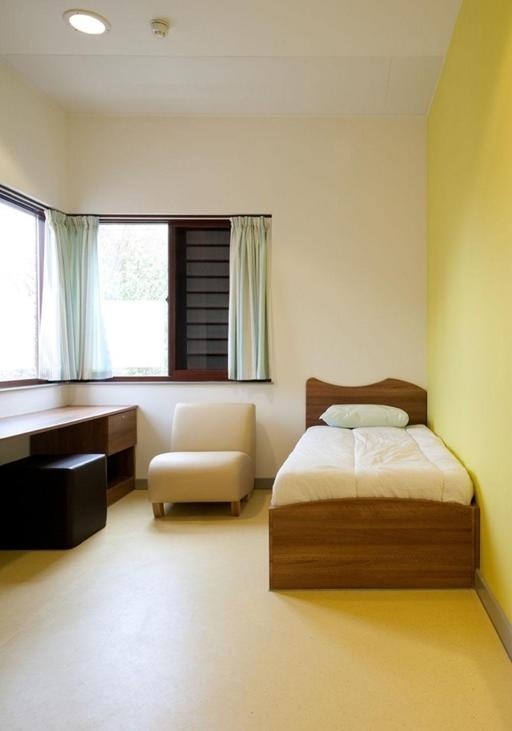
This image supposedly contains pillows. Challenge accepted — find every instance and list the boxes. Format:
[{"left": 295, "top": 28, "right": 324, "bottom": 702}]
[{"left": 316, "top": 403, "right": 410, "bottom": 430}]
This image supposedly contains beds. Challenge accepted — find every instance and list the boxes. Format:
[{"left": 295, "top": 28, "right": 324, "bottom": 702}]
[{"left": 268, "top": 376, "right": 481, "bottom": 590}]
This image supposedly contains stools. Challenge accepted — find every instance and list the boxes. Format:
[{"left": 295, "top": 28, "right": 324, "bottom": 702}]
[{"left": 2, "top": 453, "right": 110, "bottom": 549}]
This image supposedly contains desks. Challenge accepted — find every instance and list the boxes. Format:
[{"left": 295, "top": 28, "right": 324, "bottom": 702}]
[{"left": 0, "top": 404, "right": 138, "bottom": 548}]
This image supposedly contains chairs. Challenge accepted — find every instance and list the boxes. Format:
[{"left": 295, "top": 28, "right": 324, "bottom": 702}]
[{"left": 144, "top": 404, "right": 256, "bottom": 522}]
